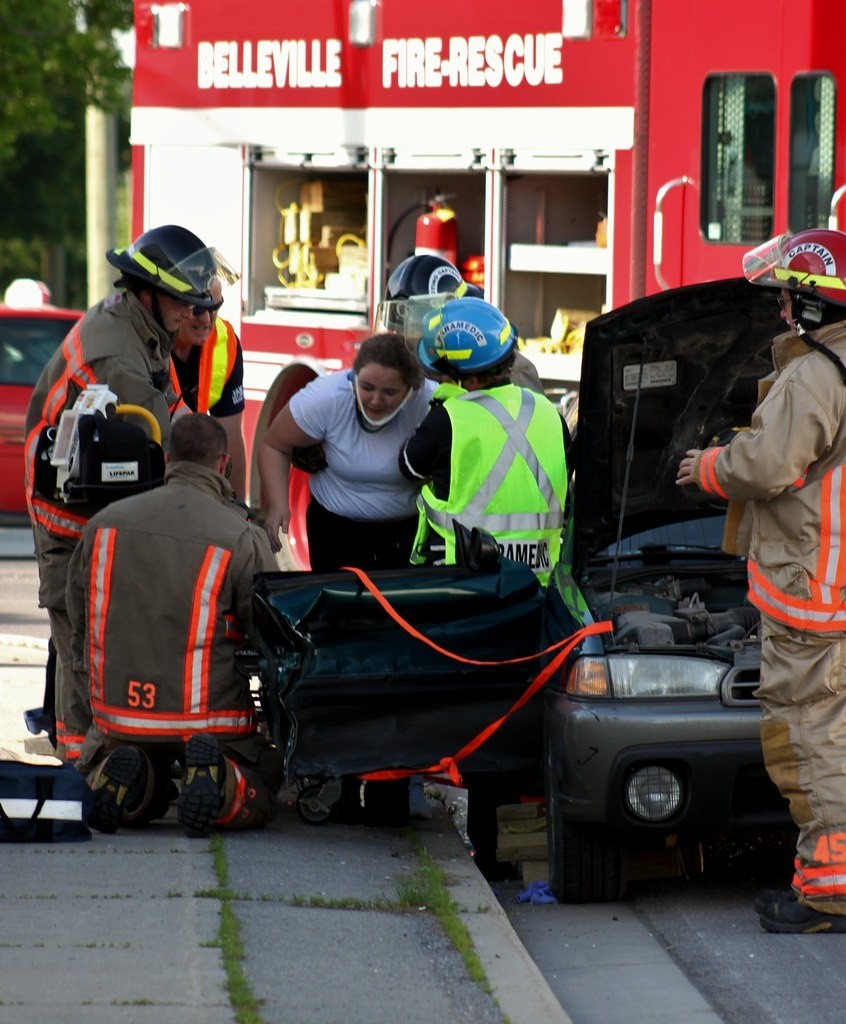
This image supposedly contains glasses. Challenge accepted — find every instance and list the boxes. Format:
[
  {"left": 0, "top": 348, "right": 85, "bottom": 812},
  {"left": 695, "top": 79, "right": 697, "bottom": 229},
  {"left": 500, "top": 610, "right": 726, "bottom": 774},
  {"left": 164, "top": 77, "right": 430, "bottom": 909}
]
[
  {"left": 777, "top": 295, "right": 790, "bottom": 309},
  {"left": 193, "top": 296, "right": 224, "bottom": 316}
]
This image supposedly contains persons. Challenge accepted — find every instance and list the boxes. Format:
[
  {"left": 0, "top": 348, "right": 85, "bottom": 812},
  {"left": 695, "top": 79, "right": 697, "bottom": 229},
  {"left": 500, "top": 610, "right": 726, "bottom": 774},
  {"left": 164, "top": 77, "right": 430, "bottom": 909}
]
[
  {"left": 675, "top": 228, "right": 846, "bottom": 935},
  {"left": 24, "top": 226, "right": 576, "bottom": 835}
]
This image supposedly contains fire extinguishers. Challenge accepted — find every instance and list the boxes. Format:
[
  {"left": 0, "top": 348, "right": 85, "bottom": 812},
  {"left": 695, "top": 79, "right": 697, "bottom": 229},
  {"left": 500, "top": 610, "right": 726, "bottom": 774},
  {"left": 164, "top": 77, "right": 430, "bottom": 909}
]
[{"left": 387, "top": 194, "right": 458, "bottom": 282}]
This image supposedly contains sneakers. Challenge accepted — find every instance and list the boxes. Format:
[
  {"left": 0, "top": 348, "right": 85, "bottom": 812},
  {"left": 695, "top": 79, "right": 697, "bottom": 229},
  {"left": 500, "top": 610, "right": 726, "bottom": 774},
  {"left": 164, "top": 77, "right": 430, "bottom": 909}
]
[{"left": 754, "top": 887, "right": 846, "bottom": 933}]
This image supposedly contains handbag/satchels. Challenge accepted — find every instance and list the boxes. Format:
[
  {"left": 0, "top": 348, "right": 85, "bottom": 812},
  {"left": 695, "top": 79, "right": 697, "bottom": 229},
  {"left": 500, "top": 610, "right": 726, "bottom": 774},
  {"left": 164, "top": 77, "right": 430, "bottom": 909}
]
[{"left": 0, "top": 747, "right": 91, "bottom": 843}]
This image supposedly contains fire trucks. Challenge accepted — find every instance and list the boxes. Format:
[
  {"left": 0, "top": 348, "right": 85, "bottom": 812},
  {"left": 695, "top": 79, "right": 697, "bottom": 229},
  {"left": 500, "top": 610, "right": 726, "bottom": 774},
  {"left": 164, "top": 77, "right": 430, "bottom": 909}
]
[{"left": 125, "top": 0, "right": 846, "bottom": 495}]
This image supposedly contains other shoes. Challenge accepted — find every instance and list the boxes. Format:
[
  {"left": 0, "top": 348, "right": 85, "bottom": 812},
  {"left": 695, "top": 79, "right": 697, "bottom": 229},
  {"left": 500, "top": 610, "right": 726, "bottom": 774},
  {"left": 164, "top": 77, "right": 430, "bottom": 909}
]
[
  {"left": 178, "top": 733, "right": 226, "bottom": 836},
  {"left": 87, "top": 746, "right": 146, "bottom": 836}
]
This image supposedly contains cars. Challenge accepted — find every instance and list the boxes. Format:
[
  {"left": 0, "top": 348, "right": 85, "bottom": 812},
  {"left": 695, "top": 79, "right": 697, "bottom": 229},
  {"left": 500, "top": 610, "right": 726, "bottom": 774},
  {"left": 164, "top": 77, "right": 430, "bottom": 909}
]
[
  {"left": 0, "top": 275, "right": 83, "bottom": 530},
  {"left": 545, "top": 279, "right": 801, "bottom": 906}
]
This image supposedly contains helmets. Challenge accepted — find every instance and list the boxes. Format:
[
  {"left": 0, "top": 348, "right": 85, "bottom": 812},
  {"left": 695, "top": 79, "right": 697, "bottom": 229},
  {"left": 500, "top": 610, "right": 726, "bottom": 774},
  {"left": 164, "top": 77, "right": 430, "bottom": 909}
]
[
  {"left": 106, "top": 224, "right": 214, "bottom": 306},
  {"left": 767, "top": 228, "right": 846, "bottom": 304},
  {"left": 381, "top": 255, "right": 484, "bottom": 331},
  {"left": 417, "top": 297, "right": 515, "bottom": 371}
]
